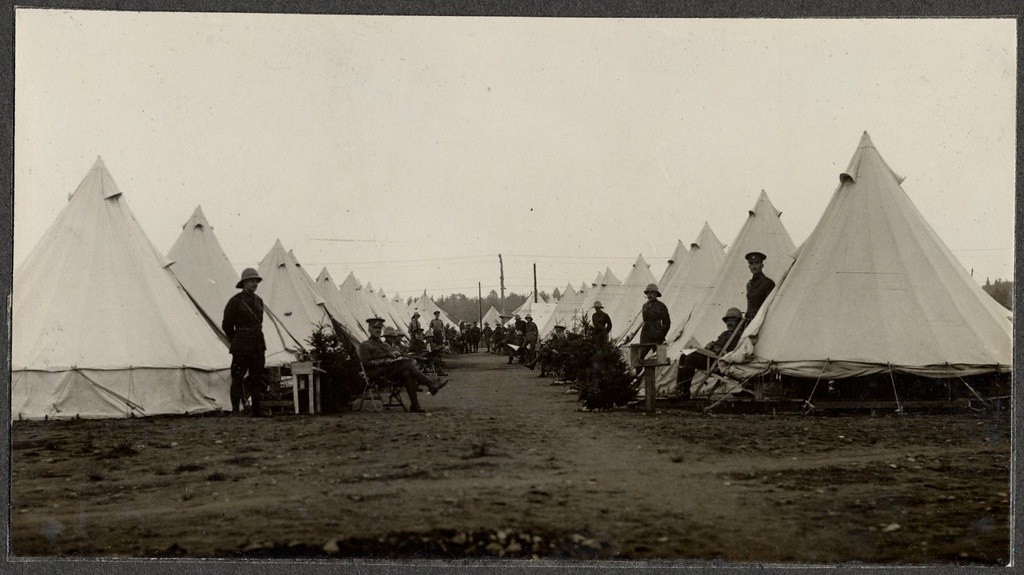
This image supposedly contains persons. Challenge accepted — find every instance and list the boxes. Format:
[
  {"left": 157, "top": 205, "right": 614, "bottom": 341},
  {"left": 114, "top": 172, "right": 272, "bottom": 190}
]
[
  {"left": 744, "top": 251, "right": 775, "bottom": 327},
  {"left": 359, "top": 318, "right": 448, "bottom": 412},
  {"left": 222, "top": 268, "right": 267, "bottom": 417},
  {"left": 384, "top": 310, "right": 566, "bottom": 391},
  {"left": 636, "top": 283, "right": 671, "bottom": 374},
  {"left": 663, "top": 307, "right": 743, "bottom": 399},
  {"left": 592, "top": 301, "right": 612, "bottom": 334}
]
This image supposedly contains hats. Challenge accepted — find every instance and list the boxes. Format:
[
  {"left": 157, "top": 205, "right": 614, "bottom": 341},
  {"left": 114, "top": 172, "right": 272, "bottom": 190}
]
[
  {"left": 434, "top": 311, "right": 440, "bottom": 314},
  {"left": 745, "top": 252, "right": 766, "bottom": 262},
  {"left": 412, "top": 312, "right": 420, "bottom": 320},
  {"left": 514, "top": 314, "right": 521, "bottom": 319},
  {"left": 412, "top": 329, "right": 424, "bottom": 333},
  {"left": 366, "top": 318, "right": 385, "bottom": 327}
]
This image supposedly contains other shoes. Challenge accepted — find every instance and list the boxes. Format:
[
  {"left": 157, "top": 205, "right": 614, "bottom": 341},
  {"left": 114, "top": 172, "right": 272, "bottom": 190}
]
[
  {"left": 429, "top": 378, "right": 449, "bottom": 396},
  {"left": 525, "top": 362, "right": 535, "bottom": 369},
  {"left": 410, "top": 404, "right": 425, "bottom": 413}
]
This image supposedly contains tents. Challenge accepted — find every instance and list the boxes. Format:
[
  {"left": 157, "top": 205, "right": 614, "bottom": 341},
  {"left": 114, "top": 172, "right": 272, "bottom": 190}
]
[
  {"left": 11, "top": 157, "right": 461, "bottom": 419},
  {"left": 478, "top": 131, "right": 1013, "bottom": 416}
]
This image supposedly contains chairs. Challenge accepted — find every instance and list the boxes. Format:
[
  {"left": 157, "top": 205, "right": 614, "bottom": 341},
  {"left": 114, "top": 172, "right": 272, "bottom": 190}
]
[
  {"left": 358, "top": 358, "right": 409, "bottom": 412},
  {"left": 692, "top": 320, "right": 747, "bottom": 399}
]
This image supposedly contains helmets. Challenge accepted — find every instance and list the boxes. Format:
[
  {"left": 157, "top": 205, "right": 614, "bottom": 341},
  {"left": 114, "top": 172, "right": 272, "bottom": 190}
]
[
  {"left": 555, "top": 322, "right": 566, "bottom": 329},
  {"left": 644, "top": 284, "right": 662, "bottom": 297},
  {"left": 236, "top": 268, "right": 262, "bottom": 289},
  {"left": 394, "top": 329, "right": 404, "bottom": 336},
  {"left": 380, "top": 327, "right": 397, "bottom": 338},
  {"left": 592, "top": 301, "right": 604, "bottom": 309},
  {"left": 525, "top": 314, "right": 533, "bottom": 320},
  {"left": 723, "top": 308, "right": 742, "bottom": 322}
]
